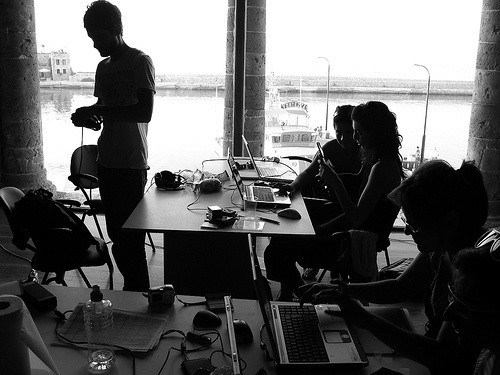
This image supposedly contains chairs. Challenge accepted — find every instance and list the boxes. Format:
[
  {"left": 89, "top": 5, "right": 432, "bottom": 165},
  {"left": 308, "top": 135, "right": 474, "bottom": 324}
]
[
  {"left": 316, "top": 171, "right": 412, "bottom": 282},
  {"left": 68, "top": 145, "right": 156, "bottom": 253},
  {"left": 0, "top": 186, "right": 115, "bottom": 292}
]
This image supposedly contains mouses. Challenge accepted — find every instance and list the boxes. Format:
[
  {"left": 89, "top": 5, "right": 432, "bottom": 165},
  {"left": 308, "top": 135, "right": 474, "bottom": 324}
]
[
  {"left": 231, "top": 318, "right": 253, "bottom": 345},
  {"left": 193, "top": 310, "right": 222, "bottom": 329},
  {"left": 278, "top": 208, "right": 301, "bottom": 219}
]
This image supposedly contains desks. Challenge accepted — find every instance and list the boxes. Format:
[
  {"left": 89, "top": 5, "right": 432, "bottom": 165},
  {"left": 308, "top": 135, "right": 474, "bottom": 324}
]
[
  {"left": 27, "top": 286, "right": 419, "bottom": 375},
  {"left": 122, "top": 155, "right": 316, "bottom": 297}
]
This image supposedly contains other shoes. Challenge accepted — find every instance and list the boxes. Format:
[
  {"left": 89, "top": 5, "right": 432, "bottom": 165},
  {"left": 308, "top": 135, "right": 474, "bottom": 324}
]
[
  {"left": 302, "top": 268, "right": 318, "bottom": 279},
  {"left": 277, "top": 286, "right": 294, "bottom": 300}
]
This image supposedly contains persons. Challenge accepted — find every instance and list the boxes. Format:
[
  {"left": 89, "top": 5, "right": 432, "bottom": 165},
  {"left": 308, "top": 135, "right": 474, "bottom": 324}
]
[
  {"left": 441, "top": 228, "right": 500, "bottom": 375},
  {"left": 71, "top": 0, "right": 156, "bottom": 293},
  {"left": 297, "top": 159, "right": 500, "bottom": 375},
  {"left": 264, "top": 101, "right": 406, "bottom": 302},
  {"left": 281, "top": 105, "right": 362, "bottom": 217}
]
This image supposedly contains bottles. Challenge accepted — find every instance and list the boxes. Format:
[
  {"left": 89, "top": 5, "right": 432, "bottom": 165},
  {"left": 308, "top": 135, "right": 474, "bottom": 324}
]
[{"left": 83, "top": 285, "right": 116, "bottom": 374}]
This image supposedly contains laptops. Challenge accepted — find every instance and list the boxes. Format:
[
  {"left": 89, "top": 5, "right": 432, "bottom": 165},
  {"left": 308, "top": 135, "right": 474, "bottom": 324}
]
[
  {"left": 224, "top": 296, "right": 241, "bottom": 375},
  {"left": 247, "top": 233, "right": 369, "bottom": 369},
  {"left": 227, "top": 135, "right": 298, "bottom": 208}
]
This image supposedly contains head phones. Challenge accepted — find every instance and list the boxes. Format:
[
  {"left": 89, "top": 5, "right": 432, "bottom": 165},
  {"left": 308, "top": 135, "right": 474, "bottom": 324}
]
[{"left": 155, "top": 170, "right": 187, "bottom": 191}]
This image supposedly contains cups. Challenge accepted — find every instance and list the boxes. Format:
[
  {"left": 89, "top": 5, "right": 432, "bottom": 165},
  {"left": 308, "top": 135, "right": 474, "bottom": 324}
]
[{"left": 243, "top": 196, "right": 258, "bottom": 218}]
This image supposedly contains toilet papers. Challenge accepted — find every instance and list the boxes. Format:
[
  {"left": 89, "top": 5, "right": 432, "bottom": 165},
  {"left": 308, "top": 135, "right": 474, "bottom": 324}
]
[{"left": 0, "top": 295, "right": 60, "bottom": 375}]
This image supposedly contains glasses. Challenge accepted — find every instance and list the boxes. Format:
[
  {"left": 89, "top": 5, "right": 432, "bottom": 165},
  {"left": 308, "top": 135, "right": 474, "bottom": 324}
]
[{"left": 401, "top": 216, "right": 438, "bottom": 234}]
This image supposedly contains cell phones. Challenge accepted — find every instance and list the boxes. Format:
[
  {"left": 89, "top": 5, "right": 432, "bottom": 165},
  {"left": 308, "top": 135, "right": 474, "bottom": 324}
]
[{"left": 317, "top": 142, "right": 326, "bottom": 163}]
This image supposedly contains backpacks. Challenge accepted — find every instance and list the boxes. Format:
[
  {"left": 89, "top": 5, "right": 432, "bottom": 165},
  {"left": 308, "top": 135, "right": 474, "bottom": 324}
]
[{"left": 13, "top": 187, "right": 96, "bottom": 262}]
